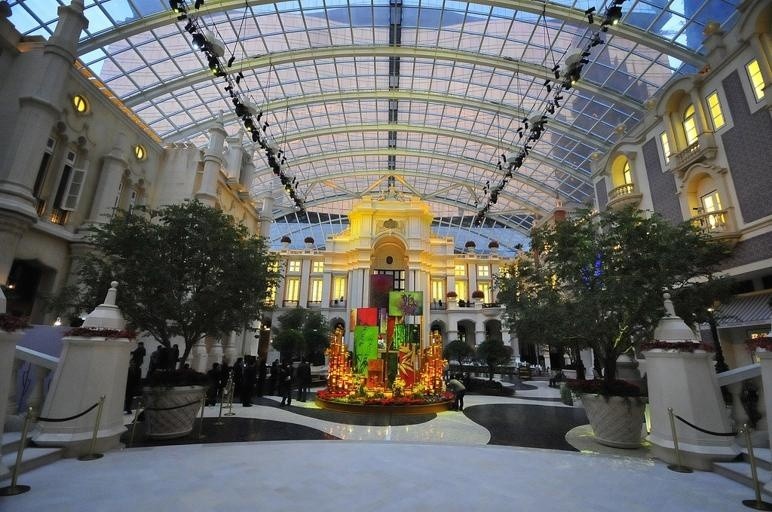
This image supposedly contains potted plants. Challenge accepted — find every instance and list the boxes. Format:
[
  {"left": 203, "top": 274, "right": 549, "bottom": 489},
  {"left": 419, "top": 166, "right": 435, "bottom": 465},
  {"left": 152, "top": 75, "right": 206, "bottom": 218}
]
[
  {"left": 487, "top": 196, "right": 744, "bottom": 449},
  {"left": 43, "top": 198, "right": 288, "bottom": 442}
]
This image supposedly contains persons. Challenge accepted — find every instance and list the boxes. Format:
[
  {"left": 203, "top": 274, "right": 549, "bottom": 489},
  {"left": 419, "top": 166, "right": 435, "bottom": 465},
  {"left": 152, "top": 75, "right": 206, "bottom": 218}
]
[
  {"left": 124, "top": 340, "right": 314, "bottom": 417},
  {"left": 548, "top": 369, "right": 562, "bottom": 388},
  {"left": 334, "top": 295, "right": 498, "bottom": 308},
  {"left": 445, "top": 378, "right": 467, "bottom": 412}
]
